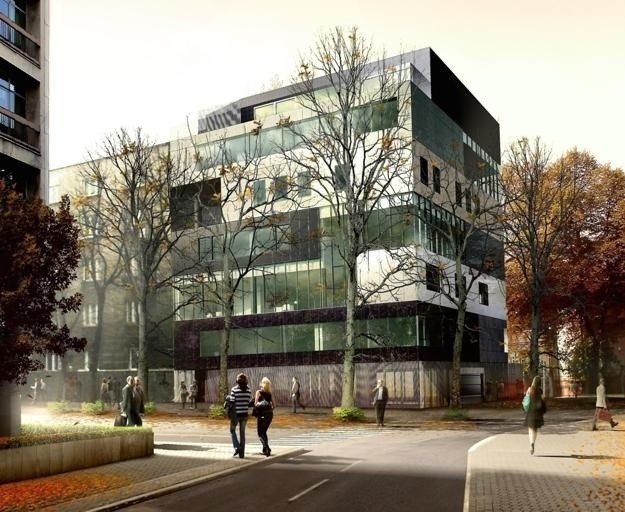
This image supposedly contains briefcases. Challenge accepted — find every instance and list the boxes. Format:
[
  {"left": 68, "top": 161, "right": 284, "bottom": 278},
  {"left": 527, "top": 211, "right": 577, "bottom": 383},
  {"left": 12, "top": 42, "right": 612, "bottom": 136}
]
[{"left": 597, "top": 406, "right": 615, "bottom": 424}]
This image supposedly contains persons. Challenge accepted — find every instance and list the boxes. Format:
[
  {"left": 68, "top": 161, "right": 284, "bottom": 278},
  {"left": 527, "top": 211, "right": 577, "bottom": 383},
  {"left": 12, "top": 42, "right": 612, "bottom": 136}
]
[
  {"left": 227, "top": 373, "right": 254, "bottom": 458},
  {"left": 523, "top": 376, "right": 546, "bottom": 455},
  {"left": 30, "top": 375, "right": 199, "bottom": 426},
  {"left": 484, "top": 375, "right": 524, "bottom": 403},
  {"left": 593, "top": 377, "right": 619, "bottom": 430},
  {"left": 254, "top": 376, "right": 277, "bottom": 458},
  {"left": 288, "top": 377, "right": 306, "bottom": 412},
  {"left": 371, "top": 378, "right": 391, "bottom": 425}
]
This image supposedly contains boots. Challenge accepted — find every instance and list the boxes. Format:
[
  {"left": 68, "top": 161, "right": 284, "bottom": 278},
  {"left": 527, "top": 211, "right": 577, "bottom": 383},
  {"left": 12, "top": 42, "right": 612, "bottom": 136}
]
[{"left": 530, "top": 442, "right": 534, "bottom": 454}]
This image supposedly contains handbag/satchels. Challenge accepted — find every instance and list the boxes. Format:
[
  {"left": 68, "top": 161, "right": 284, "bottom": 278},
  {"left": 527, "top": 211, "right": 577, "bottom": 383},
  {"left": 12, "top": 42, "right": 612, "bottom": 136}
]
[
  {"left": 523, "top": 392, "right": 530, "bottom": 412},
  {"left": 252, "top": 401, "right": 269, "bottom": 418},
  {"left": 114, "top": 413, "right": 128, "bottom": 426}
]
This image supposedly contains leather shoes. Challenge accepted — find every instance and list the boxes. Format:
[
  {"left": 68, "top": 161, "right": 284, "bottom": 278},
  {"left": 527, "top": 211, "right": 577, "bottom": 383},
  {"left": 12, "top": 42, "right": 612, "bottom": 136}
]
[
  {"left": 258, "top": 450, "right": 264, "bottom": 453},
  {"left": 265, "top": 450, "right": 270, "bottom": 456},
  {"left": 238, "top": 451, "right": 245, "bottom": 459},
  {"left": 233, "top": 448, "right": 242, "bottom": 456}
]
